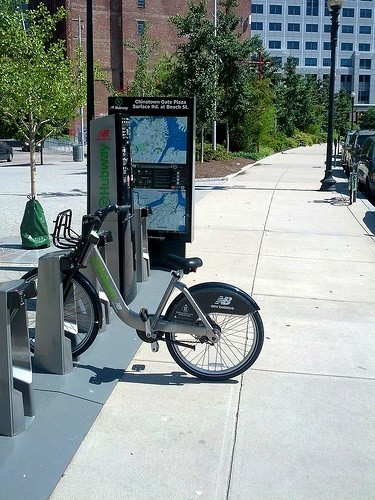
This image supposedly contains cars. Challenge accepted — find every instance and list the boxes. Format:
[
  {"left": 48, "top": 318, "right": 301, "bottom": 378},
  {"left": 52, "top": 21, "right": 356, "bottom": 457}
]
[
  {"left": 22, "top": 138, "right": 42, "bottom": 152},
  {"left": 356, "top": 136, "right": 375, "bottom": 195},
  {"left": 0, "top": 141, "right": 14, "bottom": 162},
  {"left": 342, "top": 131, "right": 375, "bottom": 177}
]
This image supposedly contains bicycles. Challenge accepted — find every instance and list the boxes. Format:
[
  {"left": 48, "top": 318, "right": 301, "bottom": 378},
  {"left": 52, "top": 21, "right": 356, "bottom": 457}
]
[
  {"left": 347, "top": 150, "right": 361, "bottom": 205},
  {"left": 20, "top": 203, "right": 264, "bottom": 381}
]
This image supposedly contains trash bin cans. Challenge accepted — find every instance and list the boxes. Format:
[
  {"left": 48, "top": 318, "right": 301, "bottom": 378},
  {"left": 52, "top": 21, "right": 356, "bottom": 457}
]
[{"left": 73, "top": 145, "right": 83, "bottom": 161}]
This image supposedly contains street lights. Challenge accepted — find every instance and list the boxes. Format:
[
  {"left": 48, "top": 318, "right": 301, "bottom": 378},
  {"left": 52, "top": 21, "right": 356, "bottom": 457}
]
[
  {"left": 350, "top": 91, "right": 356, "bottom": 130},
  {"left": 320, "top": 0, "right": 345, "bottom": 192}
]
